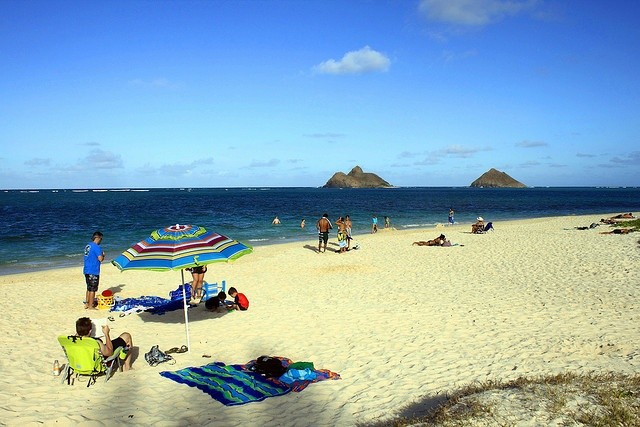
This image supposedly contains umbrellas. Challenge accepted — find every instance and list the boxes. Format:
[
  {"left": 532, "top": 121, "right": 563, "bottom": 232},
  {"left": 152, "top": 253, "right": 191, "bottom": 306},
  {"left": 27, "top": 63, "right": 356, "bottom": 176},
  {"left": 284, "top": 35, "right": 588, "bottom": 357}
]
[{"left": 110, "top": 220, "right": 256, "bottom": 356}]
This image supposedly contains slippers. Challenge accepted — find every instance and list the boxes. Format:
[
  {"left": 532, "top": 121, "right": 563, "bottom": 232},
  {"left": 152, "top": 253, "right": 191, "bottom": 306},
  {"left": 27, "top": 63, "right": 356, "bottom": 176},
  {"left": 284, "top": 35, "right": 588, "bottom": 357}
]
[
  {"left": 165, "top": 348, "right": 179, "bottom": 354},
  {"left": 176, "top": 345, "right": 188, "bottom": 353}
]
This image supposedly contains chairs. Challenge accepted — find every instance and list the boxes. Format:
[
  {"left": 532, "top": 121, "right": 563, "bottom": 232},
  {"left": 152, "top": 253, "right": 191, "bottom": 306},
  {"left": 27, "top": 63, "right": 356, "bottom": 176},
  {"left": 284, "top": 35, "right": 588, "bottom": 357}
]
[
  {"left": 57, "top": 335, "right": 132, "bottom": 387},
  {"left": 476, "top": 222, "right": 494, "bottom": 235}
]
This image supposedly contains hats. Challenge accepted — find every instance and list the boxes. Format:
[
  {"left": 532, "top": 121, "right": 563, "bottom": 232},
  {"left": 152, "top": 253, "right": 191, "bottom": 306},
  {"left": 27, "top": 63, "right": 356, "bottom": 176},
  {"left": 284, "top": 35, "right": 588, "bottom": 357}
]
[{"left": 477, "top": 217, "right": 483, "bottom": 221}]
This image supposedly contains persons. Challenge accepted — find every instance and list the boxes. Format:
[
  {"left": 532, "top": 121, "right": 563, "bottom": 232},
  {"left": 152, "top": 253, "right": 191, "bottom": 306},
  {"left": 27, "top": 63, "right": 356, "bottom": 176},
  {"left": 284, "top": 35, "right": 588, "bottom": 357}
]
[
  {"left": 272, "top": 216, "right": 281, "bottom": 224},
  {"left": 185, "top": 265, "right": 207, "bottom": 300},
  {"left": 371, "top": 213, "right": 378, "bottom": 234},
  {"left": 345, "top": 215, "right": 353, "bottom": 250},
  {"left": 471, "top": 216, "right": 484, "bottom": 233},
  {"left": 73, "top": 315, "right": 136, "bottom": 374},
  {"left": 315, "top": 214, "right": 333, "bottom": 254},
  {"left": 612, "top": 213, "right": 634, "bottom": 218},
  {"left": 598, "top": 229, "right": 631, "bottom": 235},
  {"left": 83, "top": 231, "right": 106, "bottom": 311},
  {"left": 335, "top": 217, "right": 347, "bottom": 253},
  {"left": 204, "top": 290, "right": 230, "bottom": 312},
  {"left": 448, "top": 207, "right": 454, "bottom": 225},
  {"left": 300, "top": 219, "right": 306, "bottom": 230},
  {"left": 385, "top": 215, "right": 390, "bottom": 227},
  {"left": 412, "top": 234, "right": 447, "bottom": 246},
  {"left": 228, "top": 286, "right": 249, "bottom": 312}
]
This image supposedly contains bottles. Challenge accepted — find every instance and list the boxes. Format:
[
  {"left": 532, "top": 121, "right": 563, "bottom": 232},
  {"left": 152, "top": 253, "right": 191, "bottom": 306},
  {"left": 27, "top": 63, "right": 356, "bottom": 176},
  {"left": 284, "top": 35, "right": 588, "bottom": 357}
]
[{"left": 52, "top": 360, "right": 60, "bottom": 376}]
